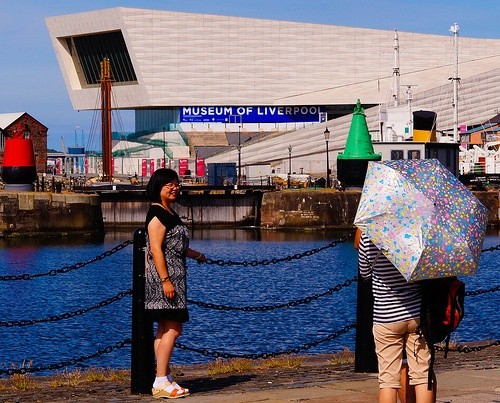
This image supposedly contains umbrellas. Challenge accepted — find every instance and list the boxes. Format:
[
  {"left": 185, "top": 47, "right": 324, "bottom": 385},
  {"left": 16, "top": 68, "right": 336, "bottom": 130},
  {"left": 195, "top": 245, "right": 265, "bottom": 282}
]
[{"left": 353, "top": 158, "right": 491, "bottom": 283}]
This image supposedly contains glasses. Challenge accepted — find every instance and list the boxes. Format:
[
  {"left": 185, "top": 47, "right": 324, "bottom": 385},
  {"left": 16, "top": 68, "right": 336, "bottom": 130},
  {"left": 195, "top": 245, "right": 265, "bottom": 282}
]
[{"left": 166, "top": 182, "right": 180, "bottom": 188}]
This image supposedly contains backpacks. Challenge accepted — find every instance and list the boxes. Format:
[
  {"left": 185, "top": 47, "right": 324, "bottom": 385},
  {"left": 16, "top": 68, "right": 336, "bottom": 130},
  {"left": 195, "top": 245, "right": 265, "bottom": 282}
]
[{"left": 419, "top": 277, "right": 466, "bottom": 390}]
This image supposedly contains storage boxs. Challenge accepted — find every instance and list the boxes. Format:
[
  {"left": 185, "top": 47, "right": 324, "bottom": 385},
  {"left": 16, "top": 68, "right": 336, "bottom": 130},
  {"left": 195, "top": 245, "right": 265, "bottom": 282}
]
[{"left": 207, "top": 163, "right": 236, "bottom": 186}]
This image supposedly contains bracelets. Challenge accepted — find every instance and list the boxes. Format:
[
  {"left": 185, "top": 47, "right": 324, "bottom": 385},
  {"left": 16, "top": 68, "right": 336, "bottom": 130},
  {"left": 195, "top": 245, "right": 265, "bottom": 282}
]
[
  {"left": 196, "top": 253, "right": 202, "bottom": 259},
  {"left": 161, "top": 277, "right": 169, "bottom": 281}
]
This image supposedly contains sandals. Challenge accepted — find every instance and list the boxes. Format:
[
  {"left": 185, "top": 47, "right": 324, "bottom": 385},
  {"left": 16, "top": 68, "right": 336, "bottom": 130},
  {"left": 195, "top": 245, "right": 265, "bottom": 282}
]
[{"left": 152, "top": 380, "right": 190, "bottom": 399}]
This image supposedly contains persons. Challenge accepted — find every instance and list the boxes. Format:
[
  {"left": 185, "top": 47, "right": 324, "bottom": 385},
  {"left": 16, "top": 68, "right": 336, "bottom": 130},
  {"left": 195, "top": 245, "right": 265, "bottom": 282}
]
[
  {"left": 144, "top": 168, "right": 208, "bottom": 398},
  {"left": 354, "top": 227, "right": 437, "bottom": 403}
]
